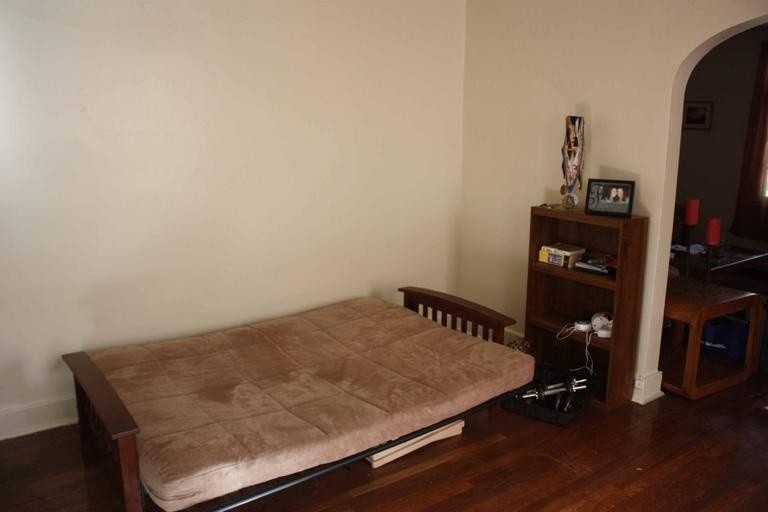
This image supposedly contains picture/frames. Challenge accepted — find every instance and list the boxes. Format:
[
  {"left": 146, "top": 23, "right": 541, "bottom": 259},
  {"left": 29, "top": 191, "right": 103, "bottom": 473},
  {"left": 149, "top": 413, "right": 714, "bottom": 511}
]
[
  {"left": 682, "top": 100, "right": 714, "bottom": 130},
  {"left": 585, "top": 178, "right": 635, "bottom": 218}
]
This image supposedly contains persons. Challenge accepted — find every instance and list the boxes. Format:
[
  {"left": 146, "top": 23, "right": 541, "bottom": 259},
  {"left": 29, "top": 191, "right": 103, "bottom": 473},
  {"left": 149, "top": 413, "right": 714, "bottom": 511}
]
[
  {"left": 600, "top": 186, "right": 617, "bottom": 202},
  {"left": 617, "top": 187, "right": 630, "bottom": 202}
]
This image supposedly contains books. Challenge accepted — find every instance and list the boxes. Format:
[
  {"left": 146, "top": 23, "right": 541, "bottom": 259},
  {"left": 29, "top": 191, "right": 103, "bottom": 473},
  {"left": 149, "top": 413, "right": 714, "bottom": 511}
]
[{"left": 537, "top": 240, "right": 611, "bottom": 275}]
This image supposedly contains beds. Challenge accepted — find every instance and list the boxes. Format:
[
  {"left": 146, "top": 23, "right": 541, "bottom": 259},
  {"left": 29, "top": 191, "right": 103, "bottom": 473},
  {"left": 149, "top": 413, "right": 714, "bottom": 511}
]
[{"left": 62, "top": 286, "right": 535, "bottom": 512}]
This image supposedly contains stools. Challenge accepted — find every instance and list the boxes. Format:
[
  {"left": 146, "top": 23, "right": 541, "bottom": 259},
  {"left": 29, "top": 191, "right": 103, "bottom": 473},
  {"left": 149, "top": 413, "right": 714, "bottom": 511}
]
[{"left": 661, "top": 274, "right": 761, "bottom": 401}]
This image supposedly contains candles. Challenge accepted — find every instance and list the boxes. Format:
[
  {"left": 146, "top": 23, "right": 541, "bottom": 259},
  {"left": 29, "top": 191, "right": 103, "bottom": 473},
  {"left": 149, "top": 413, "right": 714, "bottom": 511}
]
[{"left": 684, "top": 198, "right": 721, "bottom": 247}]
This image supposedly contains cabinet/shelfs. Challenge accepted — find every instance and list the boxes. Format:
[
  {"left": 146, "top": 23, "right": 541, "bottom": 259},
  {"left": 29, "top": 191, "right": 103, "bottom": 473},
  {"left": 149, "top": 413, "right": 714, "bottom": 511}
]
[{"left": 524, "top": 203, "right": 650, "bottom": 413}]
